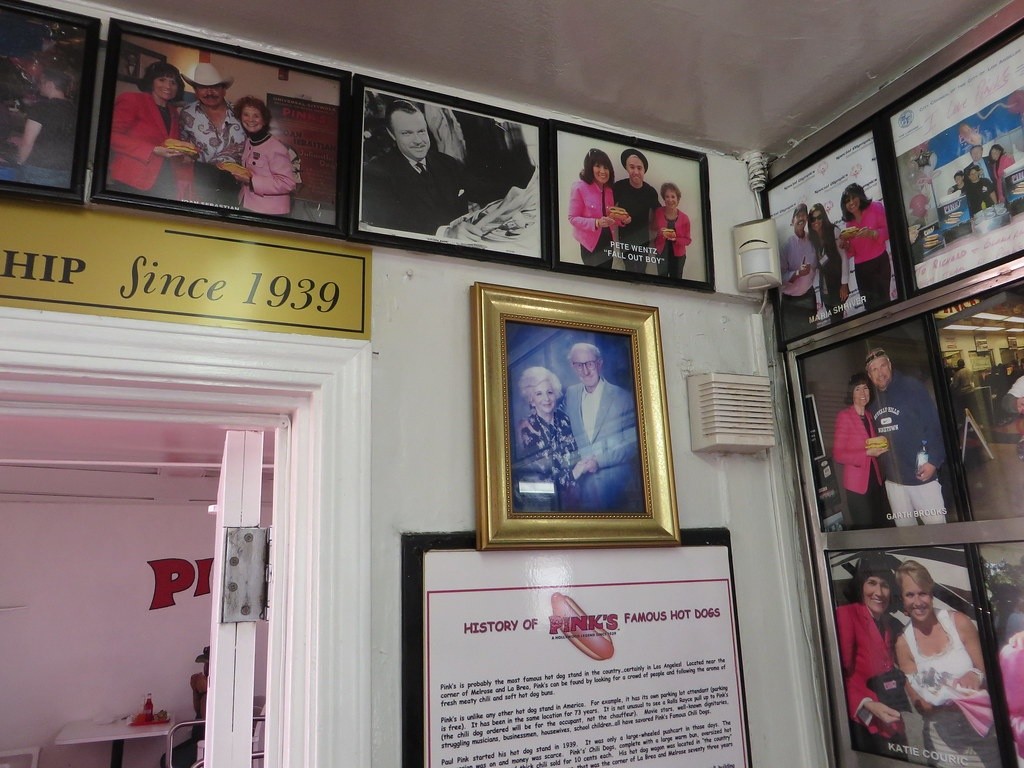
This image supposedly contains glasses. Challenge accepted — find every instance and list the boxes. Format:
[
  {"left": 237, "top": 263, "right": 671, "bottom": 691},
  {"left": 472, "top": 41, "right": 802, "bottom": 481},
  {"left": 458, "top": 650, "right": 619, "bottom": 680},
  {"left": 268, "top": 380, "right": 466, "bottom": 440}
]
[
  {"left": 811, "top": 214, "right": 823, "bottom": 222},
  {"left": 858, "top": 552, "right": 879, "bottom": 568},
  {"left": 589, "top": 148, "right": 600, "bottom": 158},
  {"left": 572, "top": 359, "right": 597, "bottom": 371},
  {"left": 865, "top": 351, "right": 884, "bottom": 364}
]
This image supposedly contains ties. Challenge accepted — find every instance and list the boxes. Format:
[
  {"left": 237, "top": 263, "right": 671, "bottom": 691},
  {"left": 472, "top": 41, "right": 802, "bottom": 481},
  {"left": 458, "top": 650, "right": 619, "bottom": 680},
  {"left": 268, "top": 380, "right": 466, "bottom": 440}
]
[{"left": 416, "top": 162, "right": 427, "bottom": 175}]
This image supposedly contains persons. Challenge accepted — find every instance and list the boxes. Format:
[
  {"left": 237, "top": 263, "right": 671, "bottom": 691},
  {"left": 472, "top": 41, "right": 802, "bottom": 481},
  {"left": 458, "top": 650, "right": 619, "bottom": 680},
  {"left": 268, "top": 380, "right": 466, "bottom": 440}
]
[
  {"left": 833, "top": 553, "right": 909, "bottom": 761},
  {"left": 832, "top": 375, "right": 895, "bottom": 528},
  {"left": 515, "top": 343, "right": 641, "bottom": 512},
  {"left": 864, "top": 348, "right": 947, "bottom": 527},
  {"left": 9, "top": 70, "right": 75, "bottom": 173},
  {"left": 568, "top": 147, "right": 691, "bottom": 278},
  {"left": 894, "top": 561, "right": 1003, "bottom": 768},
  {"left": 363, "top": 89, "right": 479, "bottom": 234},
  {"left": 942, "top": 356, "right": 1024, "bottom": 461},
  {"left": 948, "top": 143, "right": 1015, "bottom": 218},
  {"left": 110, "top": 61, "right": 301, "bottom": 217},
  {"left": 160, "top": 646, "right": 211, "bottom": 768},
  {"left": 777, "top": 183, "right": 891, "bottom": 340}
]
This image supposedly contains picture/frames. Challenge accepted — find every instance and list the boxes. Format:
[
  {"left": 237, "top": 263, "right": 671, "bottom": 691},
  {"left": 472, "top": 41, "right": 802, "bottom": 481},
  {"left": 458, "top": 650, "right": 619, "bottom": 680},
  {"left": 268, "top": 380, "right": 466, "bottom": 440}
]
[
  {"left": 760, "top": 113, "right": 1023, "bottom": 768},
  {"left": 343, "top": 72, "right": 552, "bottom": 273},
  {"left": 546, "top": 116, "right": 716, "bottom": 295},
  {"left": 0, "top": 0, "right": 101, "bottom": 210},
  {"left": 466, "top": 280, "right": 681, "bottom": 550},
  {"left": 90, "top": 16, "right": 351, "bottom": 243}
]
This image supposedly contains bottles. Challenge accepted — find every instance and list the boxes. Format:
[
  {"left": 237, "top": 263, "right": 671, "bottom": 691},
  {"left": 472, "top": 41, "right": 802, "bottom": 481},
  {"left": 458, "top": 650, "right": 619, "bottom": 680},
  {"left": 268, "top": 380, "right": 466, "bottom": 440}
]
[
  {"left": 144, "top": 693, "right": 154, "bottom": 721},
  {"left": 916, "top": 440, "right": 929, "bottom": 477}
]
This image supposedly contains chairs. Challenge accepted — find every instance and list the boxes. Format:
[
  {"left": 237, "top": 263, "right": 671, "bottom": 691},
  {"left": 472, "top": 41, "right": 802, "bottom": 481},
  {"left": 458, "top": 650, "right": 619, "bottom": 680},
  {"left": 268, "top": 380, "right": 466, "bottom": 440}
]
[{"left": 164, "top": 695, "right": 267, "bottom": 767}]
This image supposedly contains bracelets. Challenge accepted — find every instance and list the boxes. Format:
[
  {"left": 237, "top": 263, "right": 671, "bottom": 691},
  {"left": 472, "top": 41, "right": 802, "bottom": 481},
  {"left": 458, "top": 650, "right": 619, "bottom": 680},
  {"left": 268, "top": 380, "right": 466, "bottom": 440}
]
[
  {"left": 596, "top": 219, "right": 599, "bottom": 228},
  {"left": 971, "top": 668, "right": 983, "bottom": 685}
]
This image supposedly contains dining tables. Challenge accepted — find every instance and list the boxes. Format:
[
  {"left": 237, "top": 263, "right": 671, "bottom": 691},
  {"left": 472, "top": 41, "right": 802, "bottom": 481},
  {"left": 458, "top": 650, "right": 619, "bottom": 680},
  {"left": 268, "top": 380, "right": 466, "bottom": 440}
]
[{"left": 53, "top": 711, "right": 176, "bottom": 767}]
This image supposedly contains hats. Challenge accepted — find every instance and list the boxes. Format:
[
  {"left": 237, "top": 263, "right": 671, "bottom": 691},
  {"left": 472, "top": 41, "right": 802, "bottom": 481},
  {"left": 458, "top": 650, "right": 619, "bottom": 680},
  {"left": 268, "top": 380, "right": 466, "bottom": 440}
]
[
  {"left": 866, "top": 670, "right": 913, "bottom": 714},
  {"left": 180, "top": 63, "right": 234, "bottom": 90},
  {"left": 793, "top": 204, "right": 807, "bottom": 218},
  {"left": 621, "top": 149, "right": 648, "bottom": 174}
]
